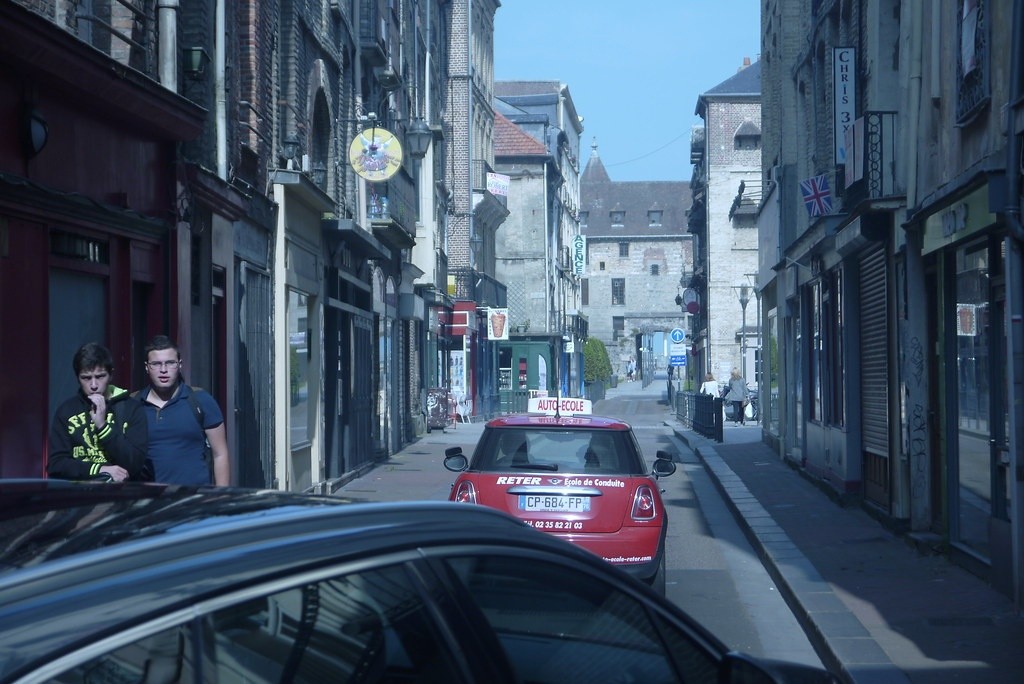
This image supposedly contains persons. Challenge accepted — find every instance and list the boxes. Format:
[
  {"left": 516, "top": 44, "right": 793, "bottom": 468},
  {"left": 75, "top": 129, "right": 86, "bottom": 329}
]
[
  {"left": 699, "top": 372, "right": 719, "bottom": 397},
  {"left": 132, "top": 336, "right": 229, "bottom": 485},
  {"left": 726, "top": 367, "right": 748, "bottom": 426},
  {"left": 626, "top": 359, "right": 636, "bottom": 382},
  {"left": 46, "top": 342, "right": 150, "bottom": 481}
]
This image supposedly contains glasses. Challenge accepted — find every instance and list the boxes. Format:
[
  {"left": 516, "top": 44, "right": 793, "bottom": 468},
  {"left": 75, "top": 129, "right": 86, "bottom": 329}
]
[{"left": 147, "top": 360, "right": 179, "bottom": 370}]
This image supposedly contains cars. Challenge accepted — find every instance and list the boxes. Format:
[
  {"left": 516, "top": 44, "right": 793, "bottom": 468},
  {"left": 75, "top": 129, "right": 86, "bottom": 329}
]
[
  {"left": 0, "top": 474, "right": 847, "bottom": 684},
  {"left": 442, "top": 412, "right": 678, "bottom": 598}
]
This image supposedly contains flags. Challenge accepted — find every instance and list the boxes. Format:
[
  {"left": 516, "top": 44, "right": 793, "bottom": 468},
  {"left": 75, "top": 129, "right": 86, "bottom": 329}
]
[{"left": 800, "top": 175, "right": 832, "bottom": 218}]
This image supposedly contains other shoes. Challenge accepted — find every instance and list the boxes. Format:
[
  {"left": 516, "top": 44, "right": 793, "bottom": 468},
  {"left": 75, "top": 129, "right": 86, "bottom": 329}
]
[{"left": 735, "top": 421, "right": 741, "bottom": 426}]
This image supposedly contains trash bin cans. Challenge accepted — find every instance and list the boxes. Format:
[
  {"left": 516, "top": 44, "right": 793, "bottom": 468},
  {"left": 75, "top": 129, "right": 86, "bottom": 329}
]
[{"left": 609, "top": 374, "right": 618, "bottom": 388}]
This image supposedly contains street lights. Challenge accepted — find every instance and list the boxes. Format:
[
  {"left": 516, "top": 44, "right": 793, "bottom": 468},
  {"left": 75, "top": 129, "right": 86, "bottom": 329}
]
[
  {"left": 732, "top": 283, "right": 755, "bottom": 386},
  {"left": 743, "top": 271, "right": 762, "bottom": 425}
]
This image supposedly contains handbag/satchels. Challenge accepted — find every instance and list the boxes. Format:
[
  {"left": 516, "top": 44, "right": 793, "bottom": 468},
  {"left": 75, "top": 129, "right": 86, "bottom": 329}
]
[
  {"left": 703, "top": 383, "right": 705, "bottom": 394},
  {"left": 742, "top": 397, "right": 753, "bottom": 421}
]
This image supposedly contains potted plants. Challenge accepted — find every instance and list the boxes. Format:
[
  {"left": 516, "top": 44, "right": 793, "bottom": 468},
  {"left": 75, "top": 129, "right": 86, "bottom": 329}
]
[{"left": 518, "top": 319, "right": 531, "bottom": 333}]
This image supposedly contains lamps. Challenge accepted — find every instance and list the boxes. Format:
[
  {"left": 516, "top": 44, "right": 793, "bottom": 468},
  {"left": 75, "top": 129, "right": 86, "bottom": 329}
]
[
  {"left": 278, "top": 132, "right": 301, "bottom": 161},
  {"left": 181, "top": 46, "right": 213, "bottom": 78},
  {"left": 728, "top": 180, "right": 773, "bottom": 221},
  {"left": 446, "top": 215, "right": 484, "bottom": 255},
  {"left": 312, "top": 161, "right": 329, "bottom": 184},
  {"left": 375, "top": 85, "right": 432, "bottom": 160}
]
